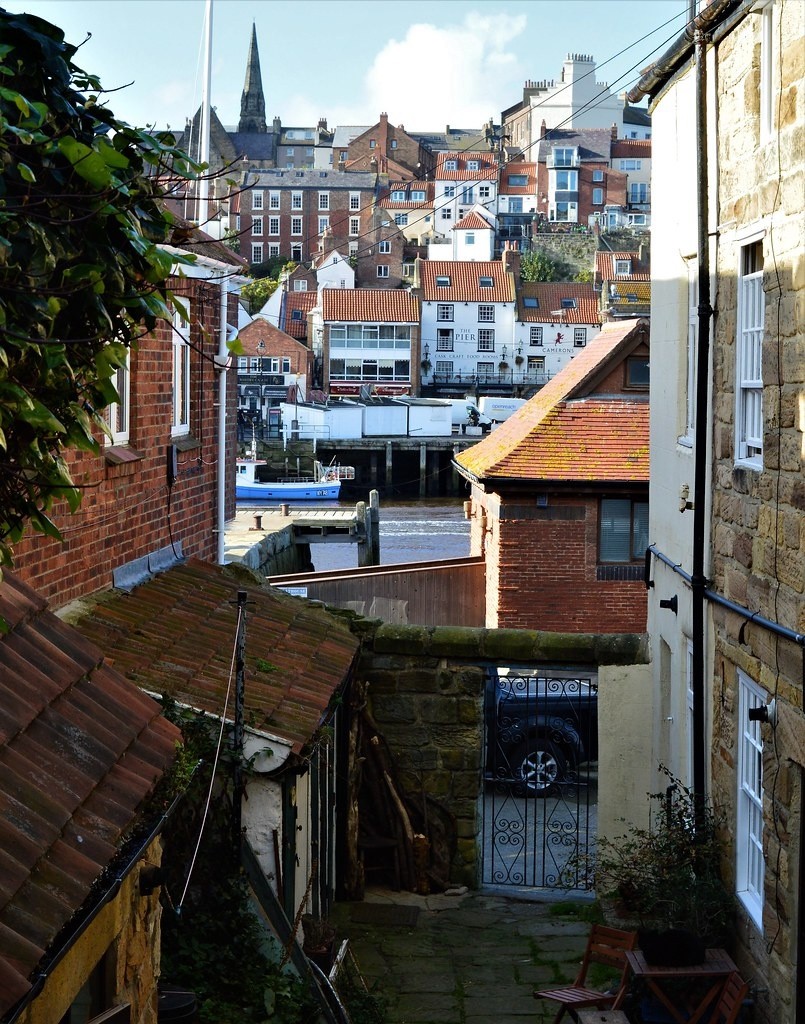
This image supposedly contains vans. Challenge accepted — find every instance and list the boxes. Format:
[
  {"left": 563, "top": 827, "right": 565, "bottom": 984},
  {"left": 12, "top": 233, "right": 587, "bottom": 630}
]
[
  {"left": 417, "top": 399, "right": 493, "bottom": 433},
  {"left": 478, "top": 397, "right": 528, "bottom": 422}
]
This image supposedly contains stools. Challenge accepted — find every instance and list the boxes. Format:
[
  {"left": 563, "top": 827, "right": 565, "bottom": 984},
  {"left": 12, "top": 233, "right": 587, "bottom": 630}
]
[{"left": 576, "top": 1010, "right": 631, "bottom": 1024}]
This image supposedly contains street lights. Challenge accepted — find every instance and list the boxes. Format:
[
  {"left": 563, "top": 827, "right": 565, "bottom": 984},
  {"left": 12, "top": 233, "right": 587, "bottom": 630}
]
[{"left": 256, "top": 339, "right": 266, "bottom": 439}]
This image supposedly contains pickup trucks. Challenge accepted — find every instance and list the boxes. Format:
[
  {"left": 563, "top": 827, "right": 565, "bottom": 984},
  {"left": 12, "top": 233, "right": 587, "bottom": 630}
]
[{"left": 486, "top": 674, "right": 599, "bottom": 794}]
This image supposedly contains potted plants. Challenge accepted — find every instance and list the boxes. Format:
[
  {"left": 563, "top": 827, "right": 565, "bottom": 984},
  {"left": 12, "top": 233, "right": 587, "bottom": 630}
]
[{"left": 498, "top": 361, "right": 509, "bottom": 371}]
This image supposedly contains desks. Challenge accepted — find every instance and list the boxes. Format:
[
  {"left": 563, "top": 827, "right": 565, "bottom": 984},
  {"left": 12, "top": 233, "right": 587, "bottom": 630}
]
[{"left": 623, "top": 949, "right": 740, "bottom": 1024}]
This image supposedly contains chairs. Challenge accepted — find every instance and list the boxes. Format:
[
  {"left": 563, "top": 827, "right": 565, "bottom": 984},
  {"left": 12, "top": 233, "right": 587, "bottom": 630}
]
[
  {"left": 532, "top": 923, "right": 638, "bottom": 1024},
  {"left": 710, "top": 966, "right": 747, "bottom": 1024}
]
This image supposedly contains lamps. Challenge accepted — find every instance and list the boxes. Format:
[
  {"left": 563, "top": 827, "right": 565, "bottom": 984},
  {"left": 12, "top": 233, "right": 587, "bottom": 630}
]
[
  {"left": 427, "top": 302, "right": 431, "bottom": 305},
  {"left": 551, "top": 323, "right": 554, "bottom": 327},
  {"left": 566, "top": 324, "right": 569, "bottom": 327},
  {"left": 591, "top": 324, "right": 596, "bottom": 328},
  {"left": 516, "top": 339, "right": 524, "bottom": 354},
  {"left": 423, "top": 342, "right": 430, "bottom": 359},
  {"left": 465, "top": 301, "right": 468, "bottom": 306},
  {"left": 500, "top": 344, "right": 508, "bottom": 360},
  {"left": 521, "top": 322, "right": 525, "bottom": 326}
]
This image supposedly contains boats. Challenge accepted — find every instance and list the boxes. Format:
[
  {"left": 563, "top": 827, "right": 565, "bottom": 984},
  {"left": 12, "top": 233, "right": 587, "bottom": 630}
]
[{"left": 235, "top": 421, "right": 355, "bottom": 500}]
